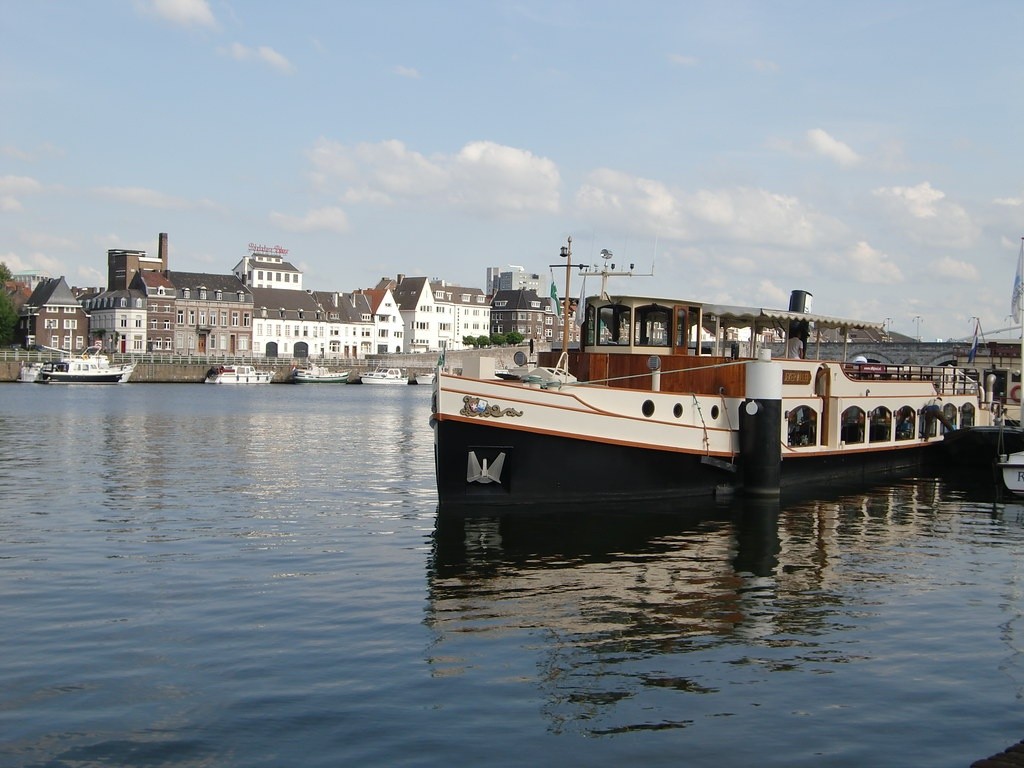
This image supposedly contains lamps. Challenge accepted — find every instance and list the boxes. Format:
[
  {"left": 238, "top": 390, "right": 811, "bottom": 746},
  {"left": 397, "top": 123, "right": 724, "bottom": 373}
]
[
  {"left": 610, "top": 264, "right": 615, "bottom": 269},
  {"left": 629, "top": 263, "right": 634, "bottom": 269},
  {"left": 600, "top": 249, "right": 612, "bottom": 267},
  {"left": 579, "top": 264, "right": 583, "bottom": 270},
  {"left": 559, "top": 247, "right": 569, "bottom": 258}
]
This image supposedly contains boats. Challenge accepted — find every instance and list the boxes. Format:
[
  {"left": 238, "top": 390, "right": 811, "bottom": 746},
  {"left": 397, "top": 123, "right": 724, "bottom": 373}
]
[
  {"left": 16, "top": 360, "right": 52, "bottom": 385},
  {"left": 426, "top": 234, "right": 1024, "bottom": 509},
  {"left": 360, "top": 362, "right": 409, "bottom": 385},
  {"left": 294, "top": 358, "right": 352, "bottom": 383},
  {"left": 204, "top": 364, "right": 278, "bottom": 384},
  {"left": 415, "top": 373, "right": 436, "bottom": 386},
  {"left": 39, "top": 344, "right": 138, "bottom": 384}
]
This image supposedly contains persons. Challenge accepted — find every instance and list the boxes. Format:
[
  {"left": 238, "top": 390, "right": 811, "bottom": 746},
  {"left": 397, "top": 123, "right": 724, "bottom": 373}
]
[{"left": 788, "top": 330, "right": 803, "bottom": 359}]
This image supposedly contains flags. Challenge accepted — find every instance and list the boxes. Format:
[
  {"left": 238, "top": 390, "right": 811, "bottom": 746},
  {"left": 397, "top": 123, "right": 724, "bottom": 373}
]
[
  {"left": 550, "top": 275, "right": 562, "bottom": 320},
  {"left": 575, "top": 280, "right": 585, "bottom": 326},
  {"left": 1011, "top": 246, "right": 1023, "bottom": 324},
  {"left": 438, "top": 346, "right": 444, "bottom": 366},
  {"left": 968, "top": 323, "right": 978, "bottom": 364}
]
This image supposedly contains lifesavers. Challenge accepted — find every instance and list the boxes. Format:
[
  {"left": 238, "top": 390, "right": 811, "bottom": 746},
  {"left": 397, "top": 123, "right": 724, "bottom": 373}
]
[
  {"left": 82, "top": 355, "right": 88, "bottom": 360},
  {"left": 1011, "top": 385, "right": 1021, "bottom": 403}
]
